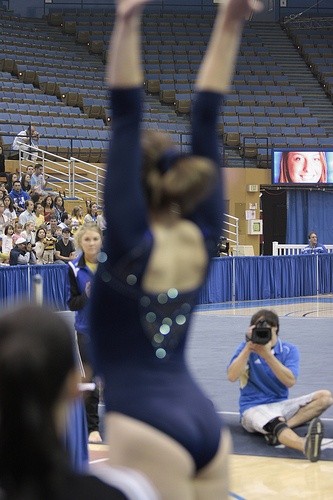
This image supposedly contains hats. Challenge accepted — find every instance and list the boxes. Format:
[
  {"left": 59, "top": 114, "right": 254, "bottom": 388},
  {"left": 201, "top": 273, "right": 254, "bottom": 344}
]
[
  {"left": 62, "top": 228, "right": 71, "bottom": 234},
  {"left": 14, "top": 237, "right": 25, "bottom": 245}
]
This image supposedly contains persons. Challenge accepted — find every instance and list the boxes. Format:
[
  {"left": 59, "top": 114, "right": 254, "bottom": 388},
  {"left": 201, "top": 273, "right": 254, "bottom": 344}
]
[
  {"left": 302, "top": 232, "right": 328, "bottom": 255},
  {"left": 13, "top": 126, "right": 41, "bottom": 161},
  {"left": 0, "top": 146, "right": 7, "bottom": 177},
  {"left": 0, "top": 164, "right": 107, "bottom": 267},
  {"left": 226, "top": 308, "right": 332, "bottom": 462},
  {"left": 278, "top": 151, "right": 328, "bottom": 184},
  {"left": 213, "top": 236, "right": 230, "bottom": 257},
  {"left": 65, "top": 221, "right": 109, "bottom": 443},
  {"left": 82, "top": 0, "right": 266, "bottom": 500},
  {"left": 0, "top": 304, "right": 161, "bottom": 500}
]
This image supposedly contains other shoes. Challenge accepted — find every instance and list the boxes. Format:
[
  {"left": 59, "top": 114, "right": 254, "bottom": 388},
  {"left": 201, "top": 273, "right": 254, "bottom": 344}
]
[
  {"left": 85, "top": 431, "right": 103, "bottom": 444},
  {"left": 265, "top": 433, "right": 280, "bottom": 446},
  {"left": 303, "top": 417, "right": 323, "bottom": 461}
]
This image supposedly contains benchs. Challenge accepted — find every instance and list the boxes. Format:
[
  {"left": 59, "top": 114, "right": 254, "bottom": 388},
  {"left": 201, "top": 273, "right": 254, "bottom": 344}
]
[{"left": 272, "top": 242, "right": 333, "bottom": 255}]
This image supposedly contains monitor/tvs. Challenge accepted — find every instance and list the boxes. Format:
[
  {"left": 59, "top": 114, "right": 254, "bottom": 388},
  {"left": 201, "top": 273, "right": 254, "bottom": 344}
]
[{"left": 271, "top": 147, "right": 333, "bottom": 188}]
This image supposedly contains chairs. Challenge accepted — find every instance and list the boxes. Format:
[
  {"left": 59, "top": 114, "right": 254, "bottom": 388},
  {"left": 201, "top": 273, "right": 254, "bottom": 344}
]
[{"left": 0, "top": 0, "right": 333, "bottom": 168}]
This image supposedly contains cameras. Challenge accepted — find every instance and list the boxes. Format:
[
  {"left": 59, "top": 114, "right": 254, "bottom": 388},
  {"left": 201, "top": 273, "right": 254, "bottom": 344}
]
[
  {"left": 27, "top": 242, "right": 36, "bottom": 247},
  {"left": 251, "top": 320, "right": 272, "bottom": 345}
]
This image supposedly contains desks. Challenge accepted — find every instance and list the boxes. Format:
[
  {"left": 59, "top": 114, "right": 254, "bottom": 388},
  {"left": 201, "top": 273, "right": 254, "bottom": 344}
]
[{"left": 0, "top": 253, "right": 333, "bottom": 310}]
